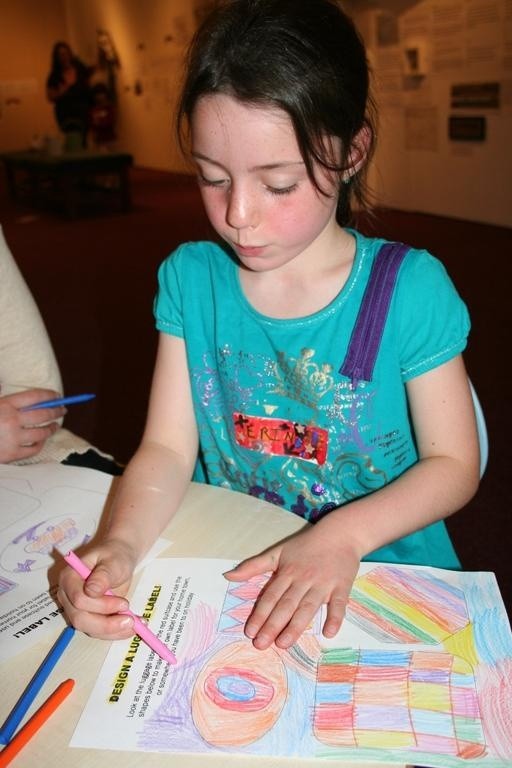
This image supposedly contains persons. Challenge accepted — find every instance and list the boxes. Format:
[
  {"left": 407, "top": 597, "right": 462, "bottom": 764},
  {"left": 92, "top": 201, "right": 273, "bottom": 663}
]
[
  {"left": 92, "top": 80, "right": 118, "bottom": 152},
  {"left": 44, "top": 40, "right": 101, "bottom": 154},
  {"left": 52, "top": 0, "right": 493, "bottom": 651},
  {"left": 0, "top": 222, "right": 72, "bottom": 468}
]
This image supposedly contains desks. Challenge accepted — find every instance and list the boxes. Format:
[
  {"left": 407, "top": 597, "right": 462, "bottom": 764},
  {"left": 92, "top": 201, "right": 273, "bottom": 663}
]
[{"left": 0, "top": 465, "right": 421, "bottom": 767}]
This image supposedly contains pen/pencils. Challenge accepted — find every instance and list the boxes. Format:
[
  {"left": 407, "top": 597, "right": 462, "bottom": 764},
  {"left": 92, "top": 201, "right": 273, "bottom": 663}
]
[
  {"left": 0, "top": 624, "right": 76, "bottom": 767},
  {"left": 24, "top": 393, "right": 96, "bottom": 414},
  {"left": 53, "top": 541, "right": 176, "bottom": 665}
]
